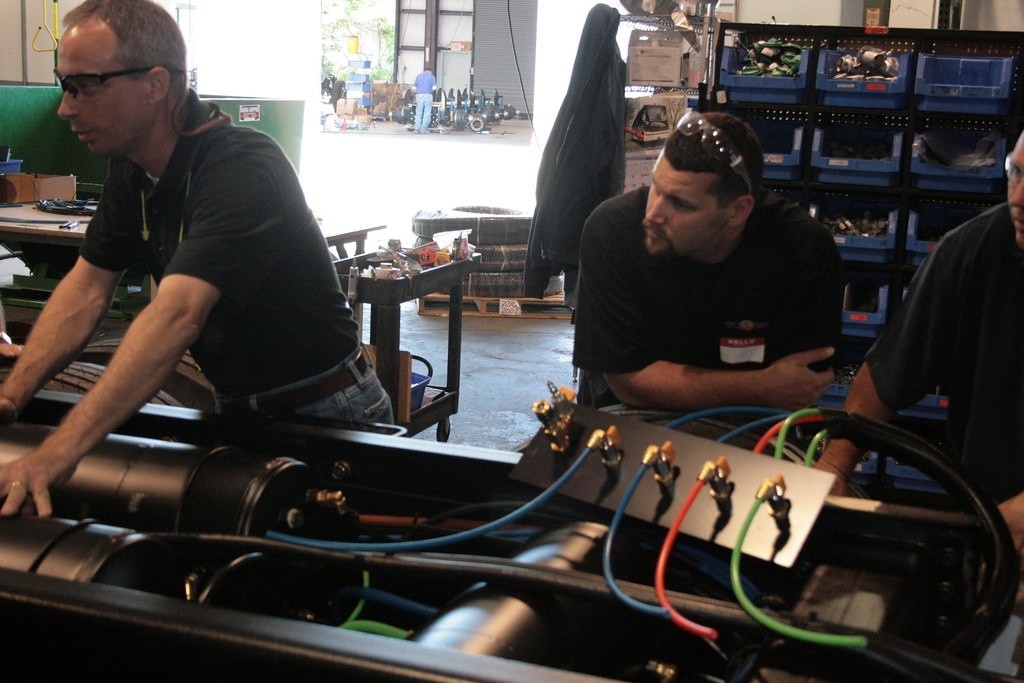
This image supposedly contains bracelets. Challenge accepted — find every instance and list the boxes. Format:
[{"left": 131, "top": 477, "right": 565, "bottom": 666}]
[{"left": 0, "top": 331, "right": 12, "bottom": 345}]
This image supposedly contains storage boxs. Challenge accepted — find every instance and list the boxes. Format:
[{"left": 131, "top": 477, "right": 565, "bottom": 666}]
[
  {"left": 0, "top": 158, "right": 77, "bottom": 203},
  {"left": 365, "top": 80, "right": 411, "bottom": 116},
  {"left": 336, "top": 98, "right": 372, "bottom": 123},
  {"left": 411, "top": 371, "right": 431, "bottom": 411}
]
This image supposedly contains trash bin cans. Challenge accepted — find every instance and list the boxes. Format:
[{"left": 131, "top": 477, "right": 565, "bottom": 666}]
[{"left": 0, "top": 159, "right": 23, "bottom": 174}]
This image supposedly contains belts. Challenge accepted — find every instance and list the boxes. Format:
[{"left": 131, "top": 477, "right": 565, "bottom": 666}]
[{"left": 216, "top": 351, "right": 368, "bottom": 413}]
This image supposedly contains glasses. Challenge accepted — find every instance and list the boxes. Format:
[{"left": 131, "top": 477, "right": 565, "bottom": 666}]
[
  {"left": 677, "top": 108, "right": 753, "bottom": 197},
  {"left": 53, "top": 65, "right": 181, "bottom": 98},
  {"left": 1004, "top": 155, "right": 1024, "bottom": 187}
]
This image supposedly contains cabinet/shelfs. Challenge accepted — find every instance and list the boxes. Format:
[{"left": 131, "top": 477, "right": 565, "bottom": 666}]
[
  {"left": 697, "top": 19, "right": 1024, "bottom": 484},
  {"left": 333, "top": 252, "right": 481, "bottom": 443},
  {"left": 0, "top": 200, "right": 388, "bottom": 342}
]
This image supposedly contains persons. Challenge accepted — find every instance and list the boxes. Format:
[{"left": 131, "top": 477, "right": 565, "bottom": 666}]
[
  {"left": 811, "top": 129, "right": 1024, "bottom": 553},
  {"left": 412, "top": 68, "right": 435, "bottom": 134},
  {"left": 571, "top": 112, "right": 848, "bottom": 411},
  {"left": 0, "top": 0, "right": 395, "bottom": 518}
]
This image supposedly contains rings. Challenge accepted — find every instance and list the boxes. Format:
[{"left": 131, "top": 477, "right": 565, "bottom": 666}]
[{"left": 7, "top": 480, "right": 27, "bottom": 493}]
